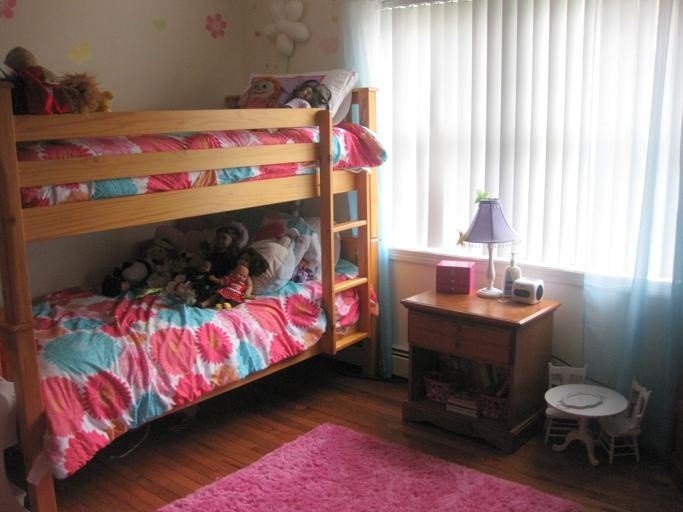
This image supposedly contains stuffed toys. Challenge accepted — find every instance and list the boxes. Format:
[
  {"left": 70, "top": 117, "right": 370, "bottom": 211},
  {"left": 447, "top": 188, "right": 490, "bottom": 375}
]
[
  {"left": 96, "top": 220, "right": 315, "bottom": 311},
  {"left": 267, "top": 80, "right": 332, "bottom": 135},
  {"left": 240, "top": 77, "right": 281, "bottom": 109},
  {"left": 3, "top": 47, "right": 115, "bottom": 118}
]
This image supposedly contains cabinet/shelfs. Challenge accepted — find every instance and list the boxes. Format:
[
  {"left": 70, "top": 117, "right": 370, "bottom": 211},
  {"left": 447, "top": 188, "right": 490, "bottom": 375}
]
[{"left": 401, "top": 287, "right": 561, "bottom": 453}]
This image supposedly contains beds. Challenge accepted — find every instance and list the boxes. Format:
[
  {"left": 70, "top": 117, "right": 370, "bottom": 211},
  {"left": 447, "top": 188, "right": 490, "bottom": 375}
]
[{"left": 0, "top": 78, "right": 382, "bottom": 512}]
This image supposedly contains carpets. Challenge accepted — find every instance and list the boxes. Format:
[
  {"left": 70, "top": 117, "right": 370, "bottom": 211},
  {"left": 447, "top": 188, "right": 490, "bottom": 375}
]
[{"left": 152, "top": 422, "right": 593, "bottom": 512}]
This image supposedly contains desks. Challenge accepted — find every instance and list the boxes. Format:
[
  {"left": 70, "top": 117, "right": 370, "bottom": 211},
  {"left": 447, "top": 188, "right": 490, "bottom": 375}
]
[{"left": 545, "top": 383, "right": 628, "bottom": 465}]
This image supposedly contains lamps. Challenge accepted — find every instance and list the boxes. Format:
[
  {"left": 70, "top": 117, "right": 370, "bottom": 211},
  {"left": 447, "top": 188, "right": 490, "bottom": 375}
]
[{"left": 461, "top": 198, "right": 517, "bottom": 299}]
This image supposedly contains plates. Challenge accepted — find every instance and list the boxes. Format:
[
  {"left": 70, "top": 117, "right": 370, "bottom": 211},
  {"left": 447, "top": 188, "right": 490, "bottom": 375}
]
[{"left": 562, "top": 391, "right": 605, "bottom": 409}]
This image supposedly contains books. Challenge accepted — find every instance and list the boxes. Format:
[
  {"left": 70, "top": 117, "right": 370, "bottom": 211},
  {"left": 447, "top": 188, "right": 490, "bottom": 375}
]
[
  {"left": 446, "top": 401, "right": 485, "bottom": 417},
  {"left": 448, "top": 384, "right": 484, "bottom": 410}
]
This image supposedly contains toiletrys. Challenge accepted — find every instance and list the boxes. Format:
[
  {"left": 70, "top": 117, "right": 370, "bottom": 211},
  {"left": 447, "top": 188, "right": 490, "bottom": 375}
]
[{"left": 504, "top": 252, "right": 521, "bottom": 297}]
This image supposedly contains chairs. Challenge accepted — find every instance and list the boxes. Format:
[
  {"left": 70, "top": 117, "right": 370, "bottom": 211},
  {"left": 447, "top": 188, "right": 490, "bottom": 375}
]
[
  {"left": 596, "top": 375, "right": 652, "bottom": 463},
  {"left": 545, "top": 361, "right": 588, "bottom": 443}
]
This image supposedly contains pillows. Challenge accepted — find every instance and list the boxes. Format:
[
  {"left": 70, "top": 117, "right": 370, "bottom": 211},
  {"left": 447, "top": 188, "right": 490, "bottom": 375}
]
[
  {"left": 236, "top": 70, "right": 357, "bottom": 125},
  {"left": 171, "top": 208, "right": 341, "bottom": 294}
]
